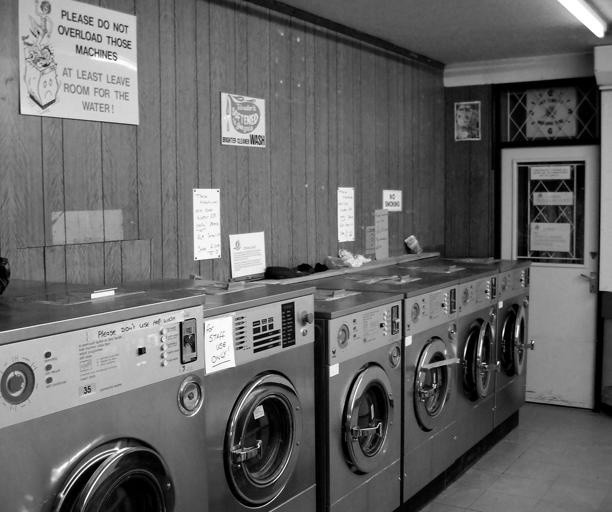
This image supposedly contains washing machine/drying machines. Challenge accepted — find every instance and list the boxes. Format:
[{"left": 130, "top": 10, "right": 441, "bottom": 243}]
[
  {"left": 169, "top": 273, "right": 321, "bottom": 512},
  {"left": 1, "top": 256, "right": 211, "bottom": 511},
  {"left": 303, "top": 259, "right": 529, "bottom": 510}
]
